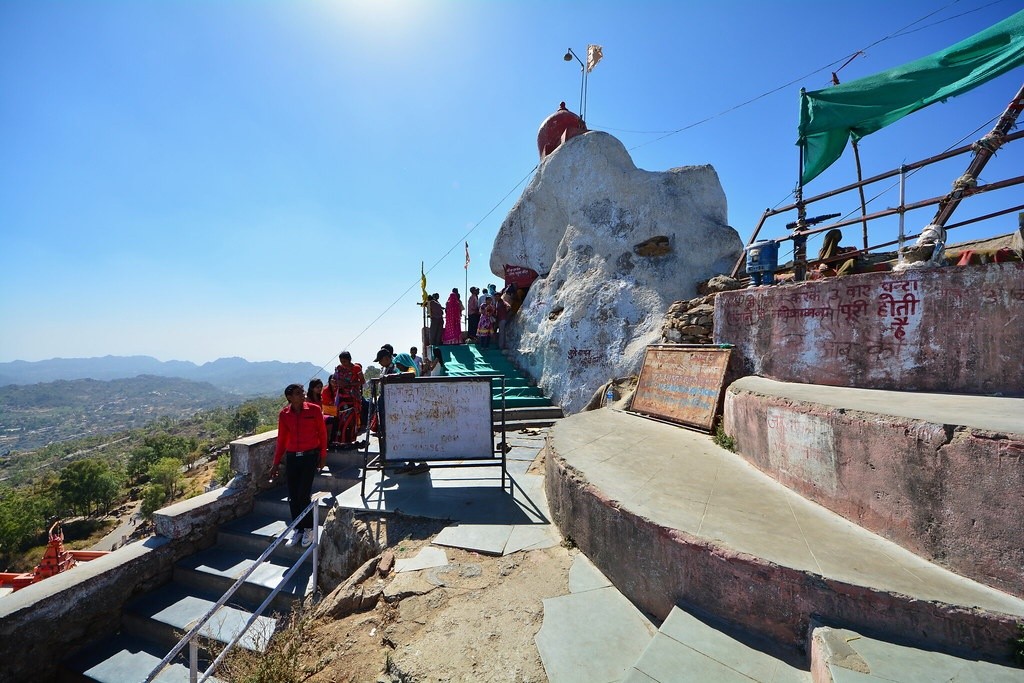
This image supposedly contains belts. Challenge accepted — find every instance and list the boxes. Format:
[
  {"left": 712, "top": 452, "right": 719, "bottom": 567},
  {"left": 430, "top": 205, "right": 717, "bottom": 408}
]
[{"left": 286, "top": 449, "right": 317, "bottom": 457}]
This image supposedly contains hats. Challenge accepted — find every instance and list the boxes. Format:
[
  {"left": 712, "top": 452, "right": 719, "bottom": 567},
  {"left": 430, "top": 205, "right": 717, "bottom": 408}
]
[{"left": 373, "top": 348, "right": 389, "bottom": 362}]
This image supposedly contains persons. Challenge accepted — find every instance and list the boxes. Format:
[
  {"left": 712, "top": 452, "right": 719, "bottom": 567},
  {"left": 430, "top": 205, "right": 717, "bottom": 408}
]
[
  {"left": 269, "top": 383, "right": 327, "bottom": 547},
  {"left": 307, "top": 281, "right": 525, "bottom": 452}
]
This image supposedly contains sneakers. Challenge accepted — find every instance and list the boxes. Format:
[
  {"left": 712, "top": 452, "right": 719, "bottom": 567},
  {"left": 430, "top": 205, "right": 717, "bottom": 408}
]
[
  {"left": 284, "top": 529, "right": 303, "bottom": 548},
  {"left": 302, "top": 528, "right": 314, "bottom": 547}
]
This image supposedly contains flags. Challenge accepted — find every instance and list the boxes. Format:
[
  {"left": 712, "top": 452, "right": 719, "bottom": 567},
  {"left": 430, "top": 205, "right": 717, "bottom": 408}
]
[
  {"left": 464, "top": 240, "right": 470, "bottom": 269},
  {"left": 420, "top": 274, "right": 429, "bottom": 306},
  {"left": 587, "top": 44, "right": 604, "bottom": 73}
]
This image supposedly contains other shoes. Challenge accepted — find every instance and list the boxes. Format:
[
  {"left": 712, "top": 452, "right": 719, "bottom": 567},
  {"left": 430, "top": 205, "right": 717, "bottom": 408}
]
[
  {"left": 394, "top": 462, "right": 415, "bottom": 474},
  {"left": 408, "top": 463, "right": 430, "bottom": 475}
]
